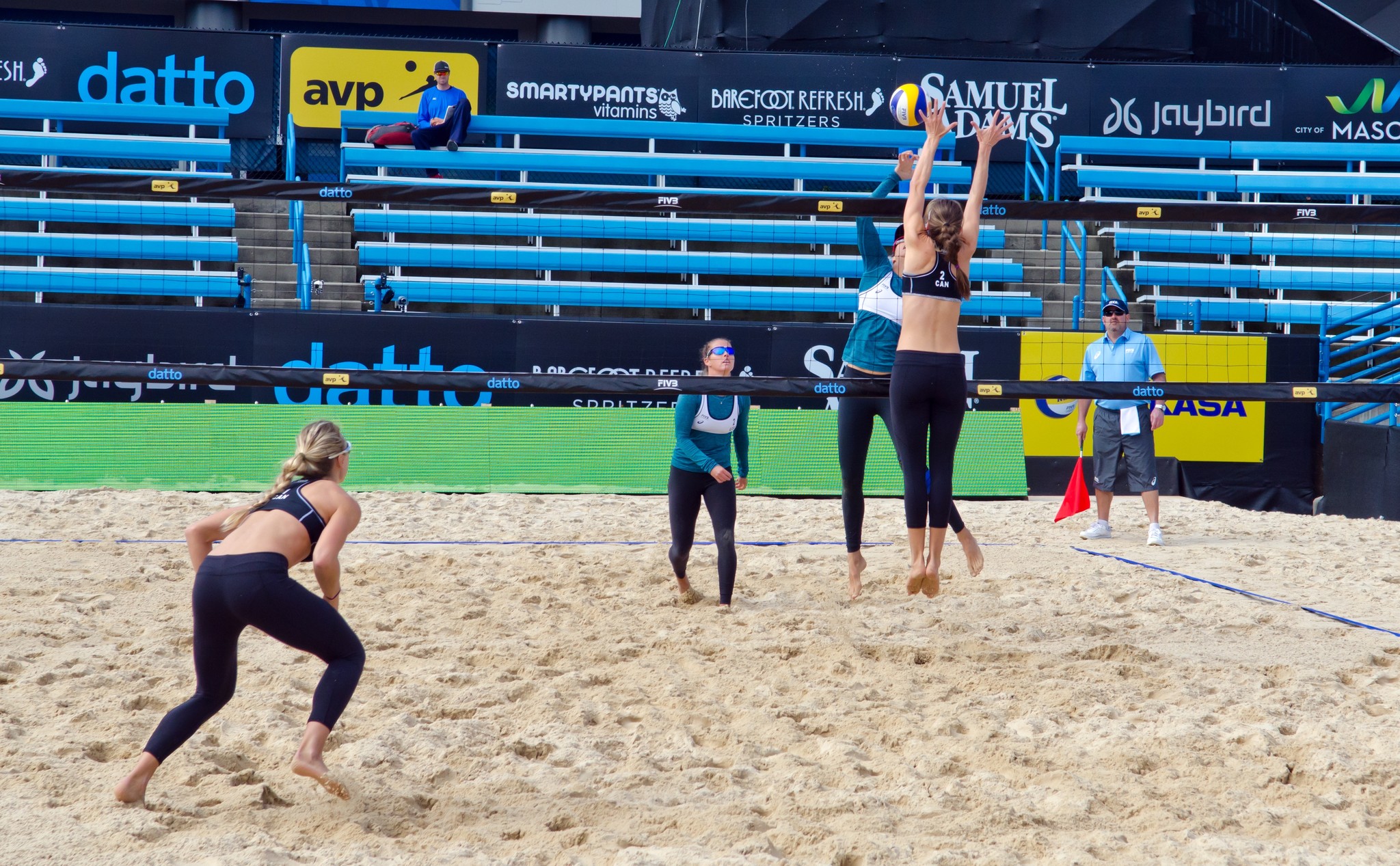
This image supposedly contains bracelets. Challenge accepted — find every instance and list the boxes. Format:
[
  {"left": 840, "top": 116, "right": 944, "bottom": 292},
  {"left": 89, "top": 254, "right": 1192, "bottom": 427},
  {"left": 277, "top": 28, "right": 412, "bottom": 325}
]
[{"left": 324, "top": 588, "right": 341, "bottom": 600}]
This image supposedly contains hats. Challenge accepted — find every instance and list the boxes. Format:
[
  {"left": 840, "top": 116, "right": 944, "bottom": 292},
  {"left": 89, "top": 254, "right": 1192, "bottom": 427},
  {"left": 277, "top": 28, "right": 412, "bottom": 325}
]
[
  {"left": 893, "top": 223, "right": 904, "bottom": 254},
  {"left": 434, "top": 60, "right": 450, "bottom": 72},
  {"left": 1102, "top": 299, "right": 1129, "bottom": 313}
]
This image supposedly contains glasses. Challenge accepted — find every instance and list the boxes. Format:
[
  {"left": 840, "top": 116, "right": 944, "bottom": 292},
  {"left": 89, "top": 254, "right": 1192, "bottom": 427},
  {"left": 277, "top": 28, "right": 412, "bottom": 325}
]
[
  {"left": 706, "top": 346, "right": 736, "bottom": 359},
  {"left": 329, "top": 441, "right": 351, "bottom": 460},
  {"left": 1103, "top": 308, "right": 1126, "bottom": 317},
  {"left": 435, "top": 71, "right": 449, "bottom": 76}
]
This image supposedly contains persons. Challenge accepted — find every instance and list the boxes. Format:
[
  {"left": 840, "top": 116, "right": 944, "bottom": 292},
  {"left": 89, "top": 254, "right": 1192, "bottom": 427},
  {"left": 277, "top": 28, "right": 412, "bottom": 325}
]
[
  {"left": 838, "top": 150, "right": 984, "bottom": 593},
  {"left": 889, "top": 99, "right": 1013, "bottom": 600},
  {"left": 115, "top": 420, "right": 367, "bottom": 806},
  {"left": 1076, "top": 299, "right": 1170, "bottom": 546},
  {"left": 667, "top": 339, "right": 751, "bottom": 606},
  {"left": 410, "top": 61, "right": 471, "bottom": 180}
]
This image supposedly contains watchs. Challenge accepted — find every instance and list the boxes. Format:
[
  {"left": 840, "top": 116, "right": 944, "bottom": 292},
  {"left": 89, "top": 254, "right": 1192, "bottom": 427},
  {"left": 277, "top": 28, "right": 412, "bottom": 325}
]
[{"left": 1155, "top": 404, "right": 1166, "bottom": 412}]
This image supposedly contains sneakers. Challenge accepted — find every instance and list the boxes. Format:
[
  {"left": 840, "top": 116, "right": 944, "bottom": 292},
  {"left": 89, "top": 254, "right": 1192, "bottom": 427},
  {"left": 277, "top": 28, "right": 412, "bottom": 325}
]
[
  {"left": 1079, "top": 523, "right": 1111, "bottom": 540},
  {"left": 1147, "top": 528, "right": 1165, "bottom": 546}
]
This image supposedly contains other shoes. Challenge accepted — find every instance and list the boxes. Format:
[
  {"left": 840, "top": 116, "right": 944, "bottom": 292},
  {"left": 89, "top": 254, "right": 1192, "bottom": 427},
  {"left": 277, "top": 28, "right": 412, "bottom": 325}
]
[{"left": 445, "top": 139, "right": 458, "bottom": 151}]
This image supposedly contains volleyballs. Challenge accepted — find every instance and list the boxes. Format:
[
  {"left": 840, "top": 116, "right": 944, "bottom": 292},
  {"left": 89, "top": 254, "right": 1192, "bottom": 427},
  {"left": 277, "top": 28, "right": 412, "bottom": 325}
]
[{"left": 889, "top": 83, "right": 932, "bottom": 127}]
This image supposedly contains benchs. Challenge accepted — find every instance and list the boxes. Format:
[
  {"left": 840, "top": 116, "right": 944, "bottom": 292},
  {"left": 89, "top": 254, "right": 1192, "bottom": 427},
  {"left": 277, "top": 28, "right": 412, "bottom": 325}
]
[
  {"left": 1058, "top": 135, "right": 1400, "bottom": 334},
  {"left": 338, "top": 110, "right": 1043, "bottom": 327},
  {"left": 0, "top": 99, "right": 242, "bottom": 307}
]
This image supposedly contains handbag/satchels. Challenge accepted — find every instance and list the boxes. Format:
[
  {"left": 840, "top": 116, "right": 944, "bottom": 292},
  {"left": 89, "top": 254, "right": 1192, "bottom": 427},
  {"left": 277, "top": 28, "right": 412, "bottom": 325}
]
[{"left": 365, "top": 122, "right": 418, "bottom": 145}]
